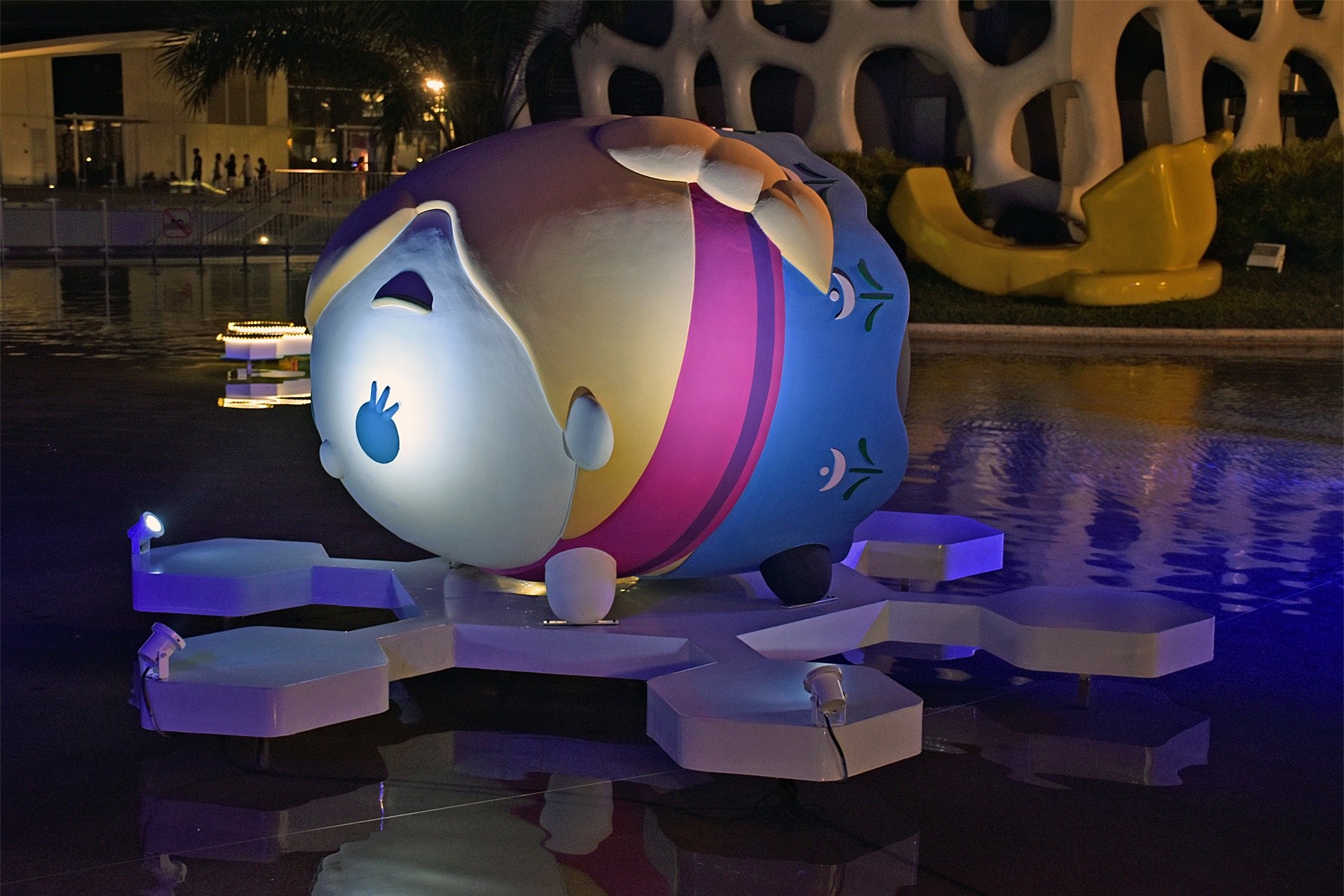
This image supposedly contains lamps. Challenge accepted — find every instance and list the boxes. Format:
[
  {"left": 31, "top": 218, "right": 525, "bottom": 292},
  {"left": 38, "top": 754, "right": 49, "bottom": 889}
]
[
  {"left": 138, "top": 622, "right": 186, "bottom": 680},
  {"left": 804, "top": 665, "right": 848, "bottom": 727},
  {"left": 1245, "top": 243, "right": 1286, "bottom": 273},
  {"left": 127, "top": 511, "right": 164, "bottom": 555}
]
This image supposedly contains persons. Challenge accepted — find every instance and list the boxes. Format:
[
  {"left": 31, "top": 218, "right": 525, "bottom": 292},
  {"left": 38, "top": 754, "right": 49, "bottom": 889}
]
[
  {"left": 191, "top": 148, "right": 202, "bottom": 185},
  {"left": 224, "top": 154, "right": 237, "bottom": 187},
  {"left": 211, "top": 153, "right": 224, "bottom": 188},
  {"left": 242, "top": 154, "right": 253, "bottom": 189},
  {"left": 254, "top": 157, "right": 268, "bottom": 185}
]
